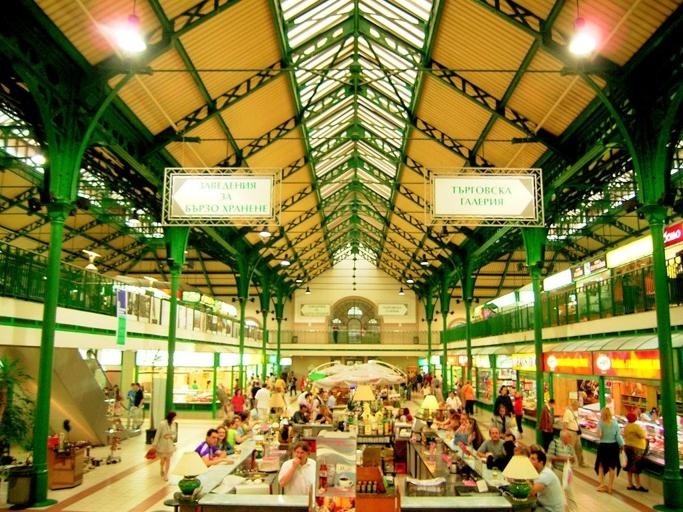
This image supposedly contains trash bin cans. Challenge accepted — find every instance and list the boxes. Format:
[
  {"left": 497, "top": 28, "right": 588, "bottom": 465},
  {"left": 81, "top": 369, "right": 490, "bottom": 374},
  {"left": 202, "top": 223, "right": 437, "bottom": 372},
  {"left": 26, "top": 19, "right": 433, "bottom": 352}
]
[{"left": 6, "top": 466, "right": 38, "bottom": 505}]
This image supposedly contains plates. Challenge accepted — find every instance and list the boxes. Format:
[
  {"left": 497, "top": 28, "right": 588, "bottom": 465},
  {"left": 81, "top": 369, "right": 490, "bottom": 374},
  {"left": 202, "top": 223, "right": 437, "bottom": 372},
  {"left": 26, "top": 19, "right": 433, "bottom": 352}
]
[{"left": 335, "top": 485, "right": 350, "bottom": 491}]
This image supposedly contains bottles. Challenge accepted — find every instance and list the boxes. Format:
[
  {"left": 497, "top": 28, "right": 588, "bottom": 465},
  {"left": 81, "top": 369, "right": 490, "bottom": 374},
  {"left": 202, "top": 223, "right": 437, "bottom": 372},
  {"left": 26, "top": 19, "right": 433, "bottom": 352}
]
[
  {"left": 318, "top": 455, "right": 328, "bottom": 492},
  {"left": 342, "top": 412, "right": 391, "bottom": 437},
  {"left": 356, "top": 481, "right": 378, "bottom": 493}
]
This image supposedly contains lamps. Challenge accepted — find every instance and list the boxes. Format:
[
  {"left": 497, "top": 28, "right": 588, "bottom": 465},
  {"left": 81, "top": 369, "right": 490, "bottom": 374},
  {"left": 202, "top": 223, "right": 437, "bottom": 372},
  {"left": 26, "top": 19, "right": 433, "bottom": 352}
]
[
  {"left": 171, "top": 449, "right": 209, "bottom": 497},
  {"left": 625, "top": 185, "right": 683, "bottom": 225},
  {"left": 501, "top": 453, "right": 539, "bottom": 502},
  {"left": 567, "top": 0, "right": 598, "bottom": 57},
  {"left": 114, "top": 1, "right": 146, "bottom": 57},
  {"left": 396, "top": 219, "right": 555, "bottom": 325}
]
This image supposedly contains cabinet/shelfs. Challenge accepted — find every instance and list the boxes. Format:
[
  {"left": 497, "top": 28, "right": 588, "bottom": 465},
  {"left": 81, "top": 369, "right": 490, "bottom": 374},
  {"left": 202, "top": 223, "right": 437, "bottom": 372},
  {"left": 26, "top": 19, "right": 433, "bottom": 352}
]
[{"left": 573, "top": 404, "right": 683, "bottom": 477}]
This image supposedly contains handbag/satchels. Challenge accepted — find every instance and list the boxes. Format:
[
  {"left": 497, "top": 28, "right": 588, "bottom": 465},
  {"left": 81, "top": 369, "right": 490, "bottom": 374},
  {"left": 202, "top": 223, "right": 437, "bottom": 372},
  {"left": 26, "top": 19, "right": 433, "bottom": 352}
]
[
  {"left": 576, "top": 429, "right": 581, "bottom": 435},
  {"left": 143, "top": 448, "right": 157, "bottom": 460}
]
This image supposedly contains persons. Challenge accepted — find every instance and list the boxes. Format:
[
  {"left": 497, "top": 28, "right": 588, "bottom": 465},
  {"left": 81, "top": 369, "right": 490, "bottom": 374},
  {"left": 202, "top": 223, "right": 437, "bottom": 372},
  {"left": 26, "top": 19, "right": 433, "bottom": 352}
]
[
  {"left": 480, "top": 307, "right": 486, "bottom": 320},
  {"left": 376, "top": 387, "right": 395, "bottom": 400},
  {"left": 154, "top": 411, "right": 177, "bottom": 480},
  {"left": 195, "top": 370, "right": 347, "bottom": 495},
  {"left": 333, "top": 324, "right": 339, "bottom": 343},
  {"left": 395, "top": 372, "right": 648, "bottom": 512},
  {"left": 102, "top": 382, "right": 145, "bottom": 430}
]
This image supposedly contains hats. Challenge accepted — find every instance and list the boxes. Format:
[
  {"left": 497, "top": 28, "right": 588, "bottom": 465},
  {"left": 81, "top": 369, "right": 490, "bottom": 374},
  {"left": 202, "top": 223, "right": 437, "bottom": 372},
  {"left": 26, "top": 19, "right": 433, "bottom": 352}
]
[{"left": 625, "top": 411, "right": 638, "bottom": 423}]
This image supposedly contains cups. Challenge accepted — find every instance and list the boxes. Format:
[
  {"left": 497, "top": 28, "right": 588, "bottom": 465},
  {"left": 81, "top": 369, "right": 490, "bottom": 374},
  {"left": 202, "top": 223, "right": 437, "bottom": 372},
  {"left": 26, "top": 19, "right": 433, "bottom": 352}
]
[{"left": 338, "top": 477, "right": 353, "bottom": 487}]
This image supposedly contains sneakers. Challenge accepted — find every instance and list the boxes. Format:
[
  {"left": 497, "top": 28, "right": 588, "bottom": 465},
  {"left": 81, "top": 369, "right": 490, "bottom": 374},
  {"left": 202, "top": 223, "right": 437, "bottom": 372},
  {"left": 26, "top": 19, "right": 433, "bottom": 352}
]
[
  {"left": 635, "top": 485, "right": 649, "bottom": 492},
  {"left": 625, "top": 484, "right": 637, "bottom": 490}
]
